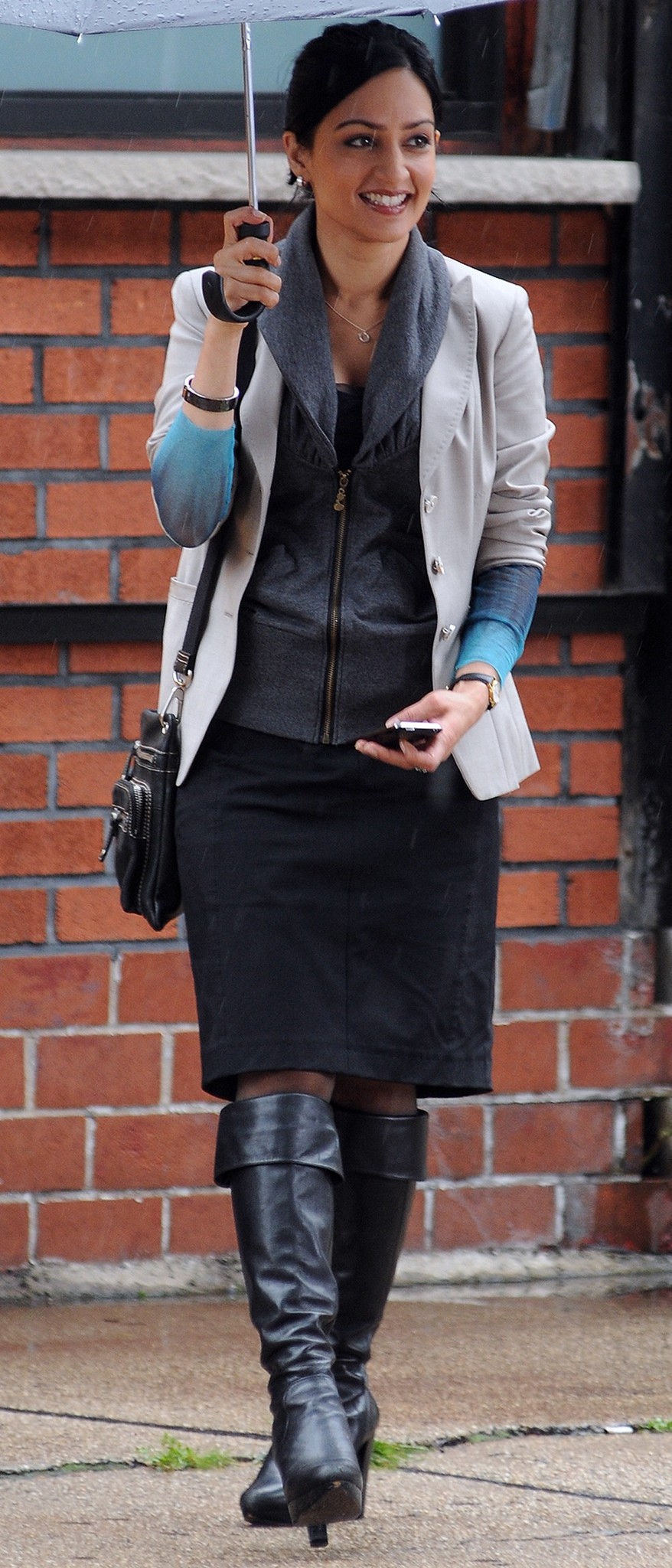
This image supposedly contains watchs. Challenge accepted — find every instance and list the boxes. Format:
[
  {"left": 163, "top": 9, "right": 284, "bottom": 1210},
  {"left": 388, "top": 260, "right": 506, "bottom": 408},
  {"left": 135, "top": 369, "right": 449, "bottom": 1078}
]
[{"left": 445, "top": 673, "right": 501, "bottom": 712}]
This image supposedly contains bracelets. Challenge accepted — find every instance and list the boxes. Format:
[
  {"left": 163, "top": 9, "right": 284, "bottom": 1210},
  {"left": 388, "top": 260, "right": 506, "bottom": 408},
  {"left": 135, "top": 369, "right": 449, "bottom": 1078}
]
[{"left": 182, "top": 375, "right": 240, "bottom": 411}]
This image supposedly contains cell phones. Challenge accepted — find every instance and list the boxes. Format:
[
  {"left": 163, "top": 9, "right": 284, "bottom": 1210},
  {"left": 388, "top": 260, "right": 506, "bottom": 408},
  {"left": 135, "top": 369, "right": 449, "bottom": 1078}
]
[{"left": 371, "top": 723, "right": 444, "bottom": 746}]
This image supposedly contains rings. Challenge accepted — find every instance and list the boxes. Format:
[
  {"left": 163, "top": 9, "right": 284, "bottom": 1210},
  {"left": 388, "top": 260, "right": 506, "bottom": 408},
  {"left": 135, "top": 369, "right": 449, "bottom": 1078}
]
[{"left": 414, "top": 767, "right": 425, "bottom": 772}]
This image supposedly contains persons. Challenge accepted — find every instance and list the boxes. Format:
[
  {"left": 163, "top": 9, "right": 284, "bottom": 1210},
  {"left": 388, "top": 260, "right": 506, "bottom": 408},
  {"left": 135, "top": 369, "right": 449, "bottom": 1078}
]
[{"left": 146, "top": 20, "right": 555, "bottom": 1545}]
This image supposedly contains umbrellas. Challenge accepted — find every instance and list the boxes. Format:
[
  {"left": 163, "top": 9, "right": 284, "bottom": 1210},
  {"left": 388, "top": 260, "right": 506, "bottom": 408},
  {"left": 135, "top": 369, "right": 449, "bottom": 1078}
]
[{"left": 0, "top": 0, "right": 505, "bottom": 324}]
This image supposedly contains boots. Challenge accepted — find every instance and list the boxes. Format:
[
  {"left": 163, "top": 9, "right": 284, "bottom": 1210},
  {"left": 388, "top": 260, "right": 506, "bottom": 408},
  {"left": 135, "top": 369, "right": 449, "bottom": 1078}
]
[
  {"left": 214, "top": 1091, "right": 364, "bottom": 1549},
  {"left": 239, "top": 1102, "right": 429, "bottom": 1526}
]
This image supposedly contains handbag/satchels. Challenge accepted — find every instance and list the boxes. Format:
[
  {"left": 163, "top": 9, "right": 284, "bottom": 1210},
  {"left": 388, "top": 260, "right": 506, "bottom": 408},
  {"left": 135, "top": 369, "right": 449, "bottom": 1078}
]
[{"left": 100, "top": 708, "right": 186, "bottom": 931}]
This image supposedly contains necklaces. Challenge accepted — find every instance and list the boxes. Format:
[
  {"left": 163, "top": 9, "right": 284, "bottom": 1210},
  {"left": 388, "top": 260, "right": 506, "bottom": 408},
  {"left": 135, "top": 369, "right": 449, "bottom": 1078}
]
[{"left": 326, "top": 301, "right": 386, "bottom": 344}]
